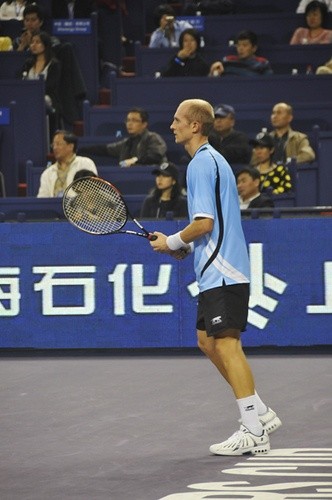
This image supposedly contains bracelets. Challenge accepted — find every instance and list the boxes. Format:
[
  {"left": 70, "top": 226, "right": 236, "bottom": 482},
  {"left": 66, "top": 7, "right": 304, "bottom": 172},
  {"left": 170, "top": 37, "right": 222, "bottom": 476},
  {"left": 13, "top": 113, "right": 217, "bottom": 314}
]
[{"left": 166, "top": 232, "right": 187, "bottom": 252}]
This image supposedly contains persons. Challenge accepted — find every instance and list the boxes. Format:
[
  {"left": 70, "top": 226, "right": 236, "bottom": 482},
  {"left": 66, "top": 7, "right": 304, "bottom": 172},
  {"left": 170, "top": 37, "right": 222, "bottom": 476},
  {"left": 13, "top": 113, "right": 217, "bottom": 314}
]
[
  {"left": 76, "top": 106, "right": 167, "bottom": 168},
  {"left": 65, "top": 170, "right": 121, "bottom": 222},
  {"left": 208, "top": 103, "right": 244, "bottom": 164},
  {"left": 148, "top": 5, "right": 193, "bottom": 49},
  {"left": 299, "top": 0, "right": 332, "bottom": 14},
  {"left": 165, "top": 29, "right": 225, "bottom": 78},
  {"left": 236, "top": 165, "right": 273, "bottom": 208},
  {"left": 223, "top": 31, "right": 273, "bottom": 80},
  {"left": 289, "top": 0, "right": 332, "bottom": 75},
  {"left": 250, "top": 128, "right": 292, "bottom": 194},
  {"left": 268, "top": 101, "right": 316, "bottom": 162},
  {"left": 15, "top": 6, "right": 59, "bottom": 51},
  {"left": 149, "top": 97, "right": 281, "bottom": 457},
  {"left": 35, "top": 129, "right": 100, "bottom": 199},
  {"left": 22, "top": 32, "right": 60, "bottom": 111},
  {"left": 139, "top": 163, "right": 187, "bottom": 219}
]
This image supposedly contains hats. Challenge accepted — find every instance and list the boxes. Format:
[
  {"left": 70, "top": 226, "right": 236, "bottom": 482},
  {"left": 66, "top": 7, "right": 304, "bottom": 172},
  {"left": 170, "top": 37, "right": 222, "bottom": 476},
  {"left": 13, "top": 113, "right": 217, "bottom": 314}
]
[
  {"left": 248, "top": 133, "right": 271, "bottom": 148},
  {"left": 214, "top": 105, "right": 233, "bottom": 117},
  {"left": 152, "top": 162, "right": 178, "bottom": 177}
]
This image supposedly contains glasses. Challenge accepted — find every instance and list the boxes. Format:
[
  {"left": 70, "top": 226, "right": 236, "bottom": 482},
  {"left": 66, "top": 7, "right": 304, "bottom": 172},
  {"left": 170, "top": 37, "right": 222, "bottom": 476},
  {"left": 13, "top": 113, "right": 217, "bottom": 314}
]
[
  {"left": 123, "top": 118, "right": 143, "bottom": 124},
  {"left": 50, "top": 140, "right": 70, "bottom": 147}
]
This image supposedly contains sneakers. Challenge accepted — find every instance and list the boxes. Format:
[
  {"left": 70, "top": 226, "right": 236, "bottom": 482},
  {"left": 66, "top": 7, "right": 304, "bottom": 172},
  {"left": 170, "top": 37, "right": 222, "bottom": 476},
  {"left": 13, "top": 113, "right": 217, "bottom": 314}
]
[
  {"left": 239, "top": 405, "right": 283, "bottom": 433},
  {"left": 210, "top": 428, "right": 271, "bottom": 457}
]
